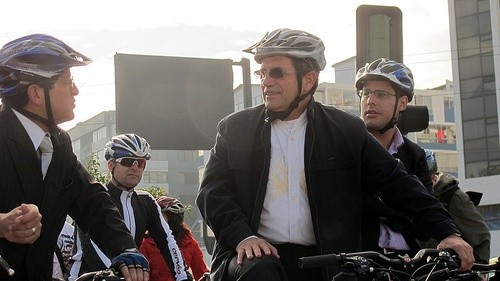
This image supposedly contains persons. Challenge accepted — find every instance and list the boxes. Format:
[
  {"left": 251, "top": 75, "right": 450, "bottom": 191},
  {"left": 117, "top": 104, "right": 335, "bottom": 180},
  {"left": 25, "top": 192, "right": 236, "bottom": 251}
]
[
  {"left": 54, "top": 132, "right": 212, "bottom": 281},
  {"left": 194, "top": 31, "right": 476, "bottom": 281},
  {"left": 355, "top": 59, "right": 493, "bottom": 281},
  {"left": 0, "top": 34, "right": 152, "bottom": 281}
]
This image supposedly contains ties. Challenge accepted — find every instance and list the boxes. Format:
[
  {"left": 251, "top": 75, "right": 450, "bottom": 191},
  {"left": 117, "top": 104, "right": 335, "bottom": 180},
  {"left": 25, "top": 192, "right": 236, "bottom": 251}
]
[{"left": 39, "top": 136, "right": 54, "bottom": 180}]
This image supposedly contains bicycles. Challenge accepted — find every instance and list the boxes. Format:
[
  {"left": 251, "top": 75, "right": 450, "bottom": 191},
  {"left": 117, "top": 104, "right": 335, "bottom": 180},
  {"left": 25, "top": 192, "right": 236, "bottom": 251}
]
[{"left": 300, "top": 246, "right": 500, "bottom": 281}]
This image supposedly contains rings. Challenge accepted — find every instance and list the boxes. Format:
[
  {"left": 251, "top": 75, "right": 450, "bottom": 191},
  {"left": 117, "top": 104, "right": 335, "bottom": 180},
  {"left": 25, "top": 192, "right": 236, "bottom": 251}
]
[{"left": 31, "top": 228, "right": 36, "bottom": 233}]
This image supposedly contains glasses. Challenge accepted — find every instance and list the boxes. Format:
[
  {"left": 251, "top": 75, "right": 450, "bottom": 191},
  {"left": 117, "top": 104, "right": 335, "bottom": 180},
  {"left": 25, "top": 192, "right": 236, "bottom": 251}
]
[
  {"left": 359, "top": 89, "right": 403, "bottom": 99},
  {"left": 35, "top": 76, "right": 74, "bottom": 90},
  {"left": 254, "top": 69, "right": 310, "bottom": 80},
  {"left": 114, "top": 158, "right": 146, "bottom": 169}
]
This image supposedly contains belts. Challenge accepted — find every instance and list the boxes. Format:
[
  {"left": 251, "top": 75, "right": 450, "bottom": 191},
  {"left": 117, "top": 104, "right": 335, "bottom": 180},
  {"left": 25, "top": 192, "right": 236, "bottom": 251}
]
[{"left": 376, "top": 247, "right": 411, "bottom": 256}]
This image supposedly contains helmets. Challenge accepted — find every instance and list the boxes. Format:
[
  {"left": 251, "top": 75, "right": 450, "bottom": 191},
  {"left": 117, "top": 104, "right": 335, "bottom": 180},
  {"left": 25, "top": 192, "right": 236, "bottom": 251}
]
[
  {"left": 156, "top": 196, "right": 186, "bottom": 214},
  {"left": 423, "top": 149, "right": 437, "bottom": 175},
  {"left": 0, "top": 34, "right": 94, "bottom": 98},
  {"left": 242, "top": 28, "right": 326, "bottom": 71},
  {"left": 354, "top": 57, "right": 414, "bottom": 103},
  {"left": 104, "top": 133, "right": 152, "bottom": 163}
]
